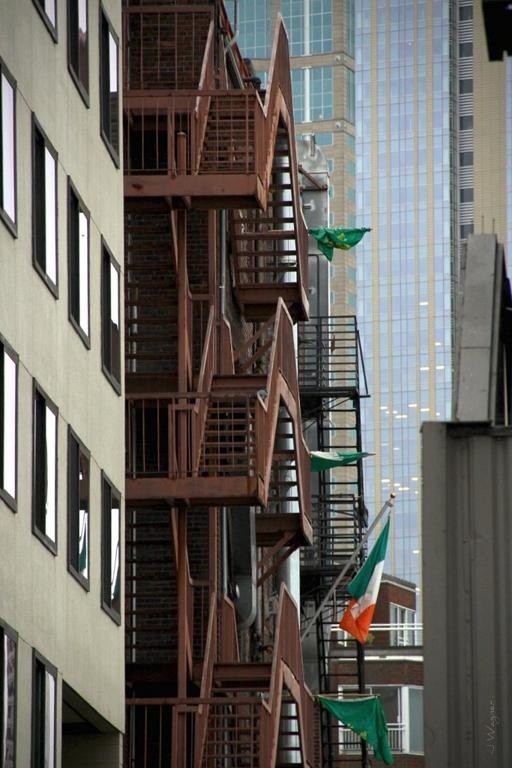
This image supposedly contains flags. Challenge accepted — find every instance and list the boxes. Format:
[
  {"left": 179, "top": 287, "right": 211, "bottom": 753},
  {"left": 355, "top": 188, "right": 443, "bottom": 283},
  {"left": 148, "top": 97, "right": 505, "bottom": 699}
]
[
  {"left": 306, "top": 226, "right": 372, "bottom": 263},
  {"left": 310, "top": 450, "right": 375, "bottom": 472},
  {"left": 318, "top": 694, "right": 394, "bottom": 768},
  {"left": 338, "top": 510, "right": 391, "bottom": 648}
]
